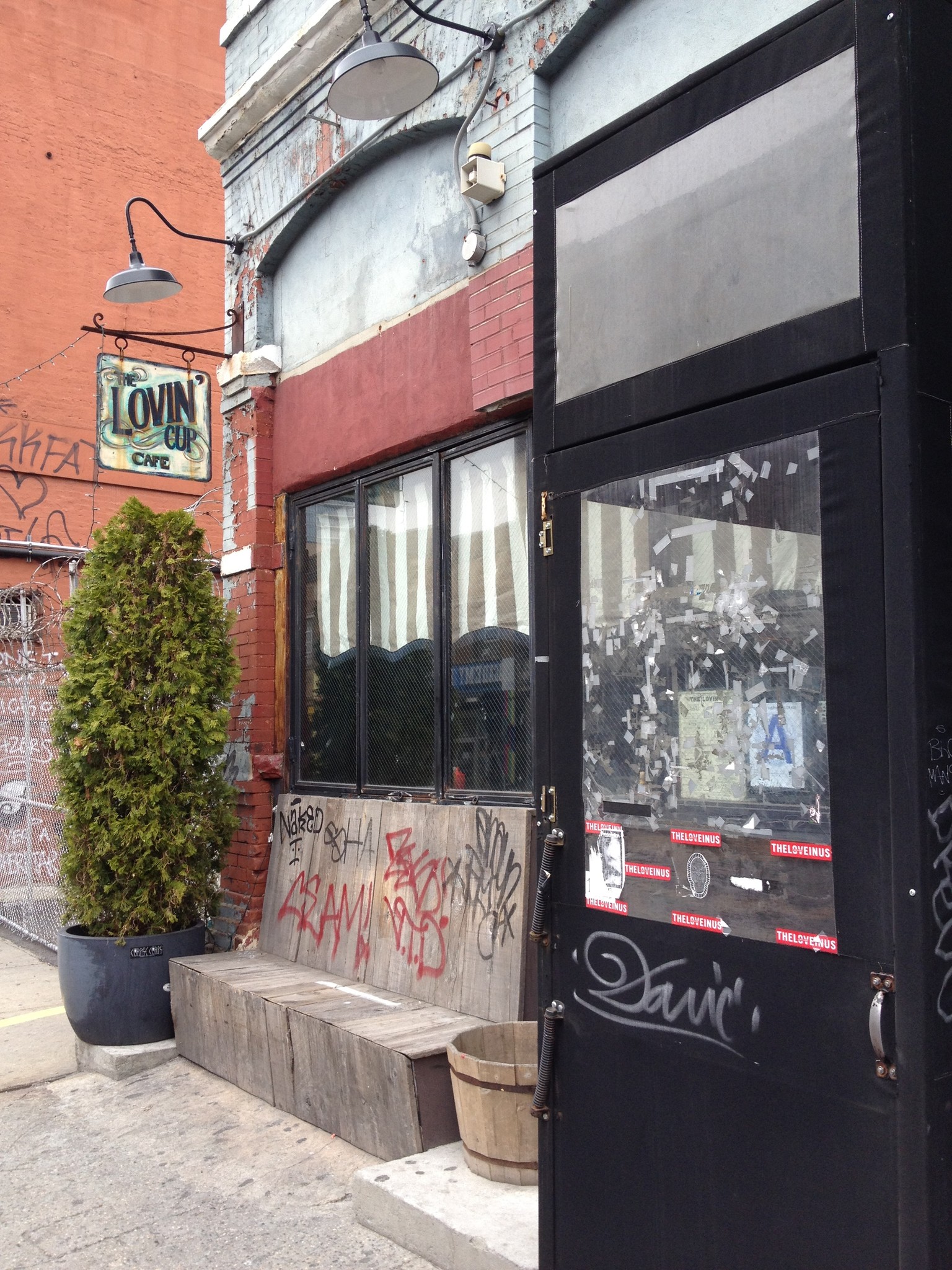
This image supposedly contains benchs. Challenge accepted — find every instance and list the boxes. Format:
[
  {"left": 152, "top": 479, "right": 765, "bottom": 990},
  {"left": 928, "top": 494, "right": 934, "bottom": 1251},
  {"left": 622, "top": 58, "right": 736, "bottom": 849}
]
[{"left": 169, "top": 792, "right": 538, "bottom": 1161}]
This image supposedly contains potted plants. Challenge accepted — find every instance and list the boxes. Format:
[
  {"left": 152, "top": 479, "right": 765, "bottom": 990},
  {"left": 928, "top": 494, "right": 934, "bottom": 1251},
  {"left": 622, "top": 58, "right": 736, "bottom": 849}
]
[{"left": 46, "top": 497, "right": 243, "bottom": 1047}]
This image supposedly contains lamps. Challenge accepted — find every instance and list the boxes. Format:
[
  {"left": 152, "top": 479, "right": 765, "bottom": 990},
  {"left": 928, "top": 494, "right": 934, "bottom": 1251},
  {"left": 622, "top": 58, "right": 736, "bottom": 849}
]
[
  {"left": 326, "top": 0, "right": 505, "bottom": 121},
  {"left": 104, "top": 196, "right": 243, "bottom": 304}
]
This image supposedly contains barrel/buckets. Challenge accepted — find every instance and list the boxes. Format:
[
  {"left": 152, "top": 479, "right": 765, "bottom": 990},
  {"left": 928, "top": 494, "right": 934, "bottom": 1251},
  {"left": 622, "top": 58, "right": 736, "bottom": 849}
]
[{"left": 446, "top": 1021, "right": 539, "bottom": 1186}]
